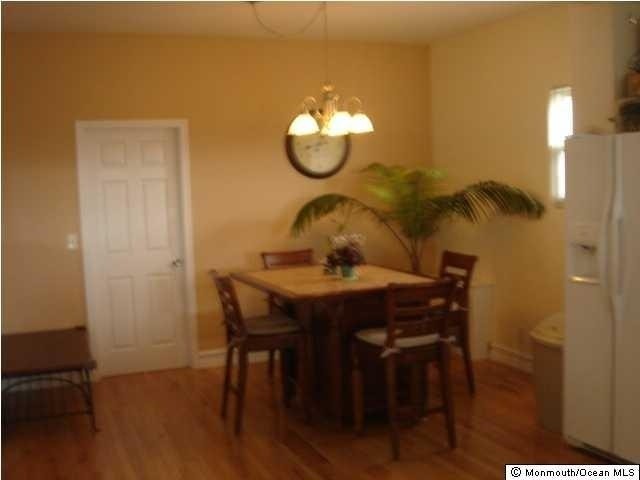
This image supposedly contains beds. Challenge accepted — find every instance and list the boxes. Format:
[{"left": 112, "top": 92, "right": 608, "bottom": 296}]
[{"left": 228, "top": 261, "right": 452, "bottom": 425}]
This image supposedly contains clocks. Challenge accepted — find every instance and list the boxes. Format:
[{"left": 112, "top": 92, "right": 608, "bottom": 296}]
[{"left": 285, "top": 109, "right": 351, "bottom": 180}]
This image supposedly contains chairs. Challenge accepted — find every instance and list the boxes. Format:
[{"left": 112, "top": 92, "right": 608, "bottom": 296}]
[
  {"left": 404, "top": 247, "right": 479, "bottom": 394},
  {"left": 348, "top": 277, "right": 459, "bottom": 460},
  {"left": 261, "top": 248, "right": 315, "bottom": 375},
  {"left": 208, "top": 268, "right": 313, "bottom": 437}
]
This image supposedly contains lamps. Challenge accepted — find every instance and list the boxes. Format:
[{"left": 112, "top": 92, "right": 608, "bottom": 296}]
[{"left": 287, "top": 1, "right": 374, "bottom": 136}]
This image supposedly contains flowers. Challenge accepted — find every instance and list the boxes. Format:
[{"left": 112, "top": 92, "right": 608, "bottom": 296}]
[{"left": 319, "top": 232, "right": 368, "bottom": 276}]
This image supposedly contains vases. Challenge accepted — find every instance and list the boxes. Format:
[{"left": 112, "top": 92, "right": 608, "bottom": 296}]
[{"left": 339, "top": 264, "right": 359, "bottom": 280}]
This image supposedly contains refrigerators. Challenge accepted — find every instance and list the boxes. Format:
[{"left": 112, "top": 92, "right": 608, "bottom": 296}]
[{"left": 566, "top": 133, "right": 639, "bottom": 463}]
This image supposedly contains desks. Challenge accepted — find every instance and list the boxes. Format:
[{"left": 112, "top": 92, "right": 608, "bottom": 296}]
[{"left": 1, "top": 325, "right": 97, "bottom": 440}]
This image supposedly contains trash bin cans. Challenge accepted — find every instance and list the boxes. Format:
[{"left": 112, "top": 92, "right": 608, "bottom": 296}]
[{"left": 529, "top": 311, "right": 564, "bottom": 434}]
